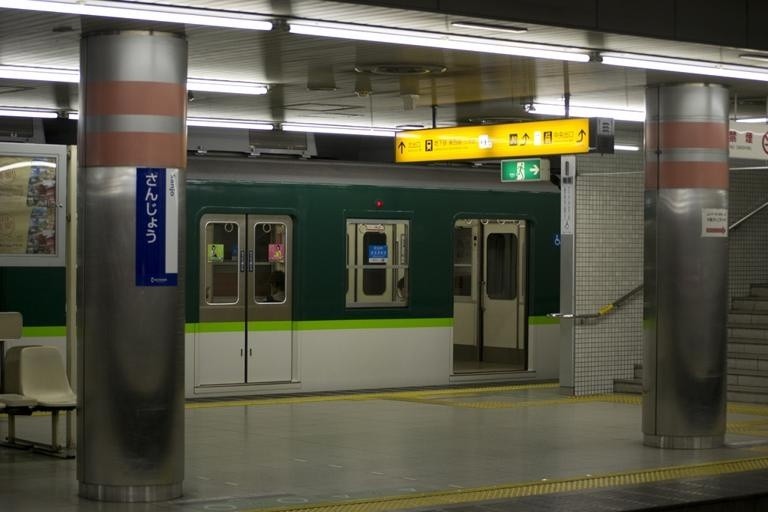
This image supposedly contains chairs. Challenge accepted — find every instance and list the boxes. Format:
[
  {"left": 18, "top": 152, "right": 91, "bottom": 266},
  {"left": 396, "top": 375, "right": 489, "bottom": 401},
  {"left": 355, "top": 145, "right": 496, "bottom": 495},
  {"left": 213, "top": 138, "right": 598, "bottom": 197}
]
[{"left": 0, "top": 345, "right": 82, "bottom": 459}]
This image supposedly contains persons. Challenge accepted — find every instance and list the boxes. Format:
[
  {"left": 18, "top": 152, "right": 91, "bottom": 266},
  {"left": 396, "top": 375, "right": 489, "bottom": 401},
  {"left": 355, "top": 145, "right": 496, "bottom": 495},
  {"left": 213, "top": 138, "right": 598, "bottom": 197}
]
[
  {"left": 261, "top": 270, "right": 285, "bottom": 303},
  {"left": 209, "top": 244, "right": 217, "bottom": 258},
  {"left": 396, "top": 277, "right": 406, "bottom": 301},
  {"left": 274, "top": 244, "right": 282, "bottom": 257}
]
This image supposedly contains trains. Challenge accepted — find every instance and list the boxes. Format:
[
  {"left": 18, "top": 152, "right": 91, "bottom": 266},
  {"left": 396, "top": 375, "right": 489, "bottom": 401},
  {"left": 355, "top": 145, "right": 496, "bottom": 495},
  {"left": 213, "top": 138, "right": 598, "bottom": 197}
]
[{"left": 0, "top": 150, "right": 562, "bottom": 405}]
